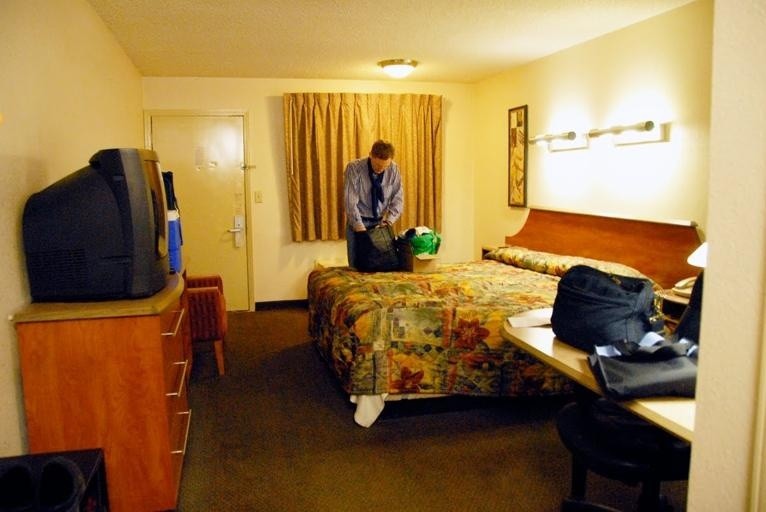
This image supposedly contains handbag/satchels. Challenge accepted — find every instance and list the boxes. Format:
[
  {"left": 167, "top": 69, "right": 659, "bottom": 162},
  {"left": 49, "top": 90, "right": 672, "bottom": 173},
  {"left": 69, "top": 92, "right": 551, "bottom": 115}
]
[
  {"left": 399, "top": 232, "right": 440, "bottom": 255},
  {"left": 590, "top": 331, "right": 697, "bottom": 400},
  {"left": 353, "top": 224, "right": 403, "bottom": 272},
  {"left": 161, "top": 171, "right": 174, "bottom": 210},
  {"left": 551, "top": 266, "right": 655, "bottom": 357}
]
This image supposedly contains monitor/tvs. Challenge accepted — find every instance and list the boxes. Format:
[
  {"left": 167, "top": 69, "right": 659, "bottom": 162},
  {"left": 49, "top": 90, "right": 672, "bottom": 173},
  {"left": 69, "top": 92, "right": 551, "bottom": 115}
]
[{"left": 21, "top": 147, "right": 169, "bottom": 302}]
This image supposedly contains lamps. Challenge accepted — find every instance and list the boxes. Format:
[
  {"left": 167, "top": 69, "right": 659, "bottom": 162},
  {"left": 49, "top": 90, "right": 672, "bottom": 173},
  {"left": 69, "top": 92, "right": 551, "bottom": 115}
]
[
  {"left": 533, "top": 131, "right": 591, "bottom": 153},
  {"left": 378, "top": 59, "right": 419, "bottom": 79},
  {"left": 589, "top": 121, "right": 669, "bottom": 146}
]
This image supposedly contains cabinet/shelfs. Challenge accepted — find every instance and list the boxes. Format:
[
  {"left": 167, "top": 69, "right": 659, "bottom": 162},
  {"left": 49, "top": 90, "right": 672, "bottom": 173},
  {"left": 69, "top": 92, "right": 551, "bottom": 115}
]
[{"left": 12, "top": 273, "right": 190, "bottom": 512}]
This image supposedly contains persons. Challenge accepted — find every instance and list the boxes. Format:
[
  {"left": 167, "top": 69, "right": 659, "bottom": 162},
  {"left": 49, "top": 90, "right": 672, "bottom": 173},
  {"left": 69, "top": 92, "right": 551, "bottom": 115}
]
[{"left": 343, "top": 139, "right": 404, "bottom": 269}]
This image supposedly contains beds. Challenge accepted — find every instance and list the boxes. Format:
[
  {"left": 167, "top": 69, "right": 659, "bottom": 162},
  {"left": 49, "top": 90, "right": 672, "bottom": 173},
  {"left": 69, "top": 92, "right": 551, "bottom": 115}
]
[{"left": 307, "top": 208, "right": 705, "bottom": 430}]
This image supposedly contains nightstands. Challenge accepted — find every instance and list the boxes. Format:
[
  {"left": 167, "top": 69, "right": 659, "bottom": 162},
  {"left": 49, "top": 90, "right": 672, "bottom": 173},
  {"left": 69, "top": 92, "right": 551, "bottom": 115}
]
[{"left": 652, "top": 287, "right": 689, "bottom": 333}]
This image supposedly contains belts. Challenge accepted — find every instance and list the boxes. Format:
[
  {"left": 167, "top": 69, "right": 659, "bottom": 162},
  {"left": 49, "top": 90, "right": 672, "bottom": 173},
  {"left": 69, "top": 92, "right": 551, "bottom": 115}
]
[{"left": 361, "top": 216, "right": 383, "bottom": 222}]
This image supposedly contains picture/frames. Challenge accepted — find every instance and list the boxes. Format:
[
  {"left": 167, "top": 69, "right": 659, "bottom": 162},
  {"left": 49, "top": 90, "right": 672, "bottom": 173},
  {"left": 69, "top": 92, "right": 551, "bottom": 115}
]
[{"left": 508, "top": 104, "right": 528, "bottom": 206}]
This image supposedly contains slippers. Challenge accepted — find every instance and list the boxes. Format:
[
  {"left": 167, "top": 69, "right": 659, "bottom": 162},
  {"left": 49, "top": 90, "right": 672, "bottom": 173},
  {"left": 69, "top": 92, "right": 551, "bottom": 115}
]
[
  {"left": 35, "top": 456, "right": 84, "bottom": 512},
  {"left": 0, "top": 459, "right": 35, "bottom": 512}
]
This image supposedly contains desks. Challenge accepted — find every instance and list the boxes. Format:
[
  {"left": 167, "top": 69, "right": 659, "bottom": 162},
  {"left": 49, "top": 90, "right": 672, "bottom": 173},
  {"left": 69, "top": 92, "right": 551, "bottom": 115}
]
[{"left": 498, "top": 307, "right": 697, "bottom": 443}]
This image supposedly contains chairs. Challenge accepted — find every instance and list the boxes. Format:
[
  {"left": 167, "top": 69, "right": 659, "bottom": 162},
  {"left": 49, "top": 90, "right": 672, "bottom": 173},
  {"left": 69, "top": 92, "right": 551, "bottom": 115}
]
[
  {"left": 182, "top": 275, "right": 227, "bottom": 377},
  {"left": 556, "top": 271, "right": 703, "bottom": 510}
]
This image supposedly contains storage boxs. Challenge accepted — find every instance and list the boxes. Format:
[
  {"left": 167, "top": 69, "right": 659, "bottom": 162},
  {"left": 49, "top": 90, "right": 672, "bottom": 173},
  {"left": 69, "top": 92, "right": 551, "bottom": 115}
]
[
  {"left": 404, "top": 243, "right": 440, "bottom": 274},
  {"left": 165, "top": 208, "right": 183, "bottom": 275}
]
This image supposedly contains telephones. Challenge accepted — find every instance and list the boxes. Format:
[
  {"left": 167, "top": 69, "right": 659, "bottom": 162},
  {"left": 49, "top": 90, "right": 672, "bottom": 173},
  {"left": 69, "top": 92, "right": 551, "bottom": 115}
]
[{"left": 672, "top": 276, "right": 697, "bottom": 298}]
